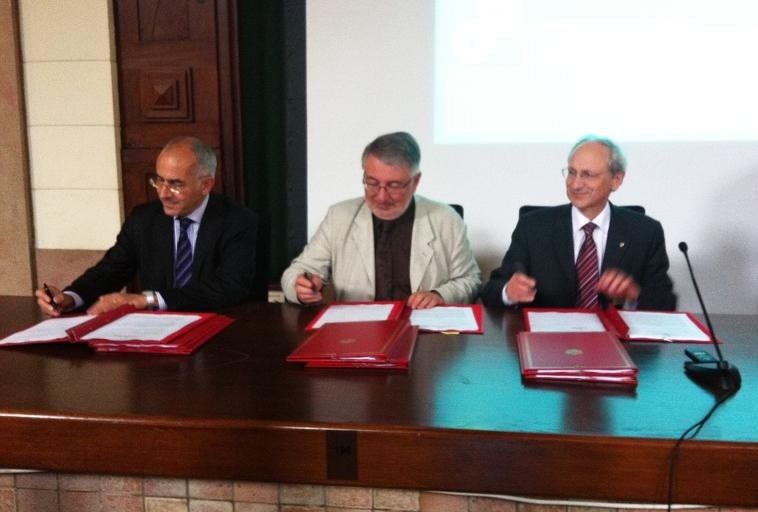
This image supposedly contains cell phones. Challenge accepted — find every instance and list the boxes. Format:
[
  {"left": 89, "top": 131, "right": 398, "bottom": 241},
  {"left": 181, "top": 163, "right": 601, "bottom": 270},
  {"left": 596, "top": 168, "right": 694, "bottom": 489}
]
[{"left": 684, "top": 347, "right": 716, "bottom": 362}]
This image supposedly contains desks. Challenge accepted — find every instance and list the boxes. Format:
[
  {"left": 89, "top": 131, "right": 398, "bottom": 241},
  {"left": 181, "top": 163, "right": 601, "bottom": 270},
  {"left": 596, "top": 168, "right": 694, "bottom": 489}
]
[{"left": 0, "top": 294, "right": 758, "bottom": 510}]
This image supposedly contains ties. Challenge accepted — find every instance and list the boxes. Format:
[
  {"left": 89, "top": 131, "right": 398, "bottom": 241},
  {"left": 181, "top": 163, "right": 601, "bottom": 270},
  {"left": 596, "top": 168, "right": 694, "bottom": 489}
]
[
  {"left": 174, "top": 213, "right": 199, "bottom": 292},
  {"left": 576, "top": 218, "right": 600, "bottom": 313},
  {"left": 377, "top": 223, "right": 394, "bottom": 301}
]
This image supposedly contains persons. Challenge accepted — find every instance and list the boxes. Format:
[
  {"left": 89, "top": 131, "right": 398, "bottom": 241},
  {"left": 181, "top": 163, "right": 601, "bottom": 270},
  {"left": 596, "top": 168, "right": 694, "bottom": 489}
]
[
  {"left": 35, "top": 136, "right": 272, "bottom": 319},
  {"left": 279, "top": 132, "right": 483, "bottom": 309},
  {"left": 481, "top": 134, "right": 678, "bottom": 311}
]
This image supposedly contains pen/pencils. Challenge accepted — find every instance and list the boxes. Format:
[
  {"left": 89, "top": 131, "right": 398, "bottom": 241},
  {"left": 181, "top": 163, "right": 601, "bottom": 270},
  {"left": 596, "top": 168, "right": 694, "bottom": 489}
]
[
  {"left": 304, "top": 272, "right": 318, "bottom": 295},
  {"left": 44, "top": 283, "right": 60, "bottom": 312}
]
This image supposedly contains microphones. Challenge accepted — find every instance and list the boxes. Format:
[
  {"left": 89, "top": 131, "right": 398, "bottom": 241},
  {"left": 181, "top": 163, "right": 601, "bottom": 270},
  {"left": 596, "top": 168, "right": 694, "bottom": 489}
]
[{"left": 678, "top": 240, "right": 742, "bottom": 395}]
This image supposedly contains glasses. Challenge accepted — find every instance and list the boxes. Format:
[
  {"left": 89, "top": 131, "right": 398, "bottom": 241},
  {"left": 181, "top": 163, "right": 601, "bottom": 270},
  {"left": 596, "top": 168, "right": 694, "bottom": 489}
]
[
  {"left": 151, "top": 175, "right": 197, "bottom": 194},
  {"left": 562, "top": 167, "right": 608, "bottom": 182},
  {"left": 363, "top": 178, "right": 411, "bottom": 197}
]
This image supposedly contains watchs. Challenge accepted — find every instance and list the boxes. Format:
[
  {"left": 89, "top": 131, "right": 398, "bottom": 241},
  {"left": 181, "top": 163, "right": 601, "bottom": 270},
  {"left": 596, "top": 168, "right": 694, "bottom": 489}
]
[{"left": 142, "top": 290, "right": 155, "bottom": 310}]
